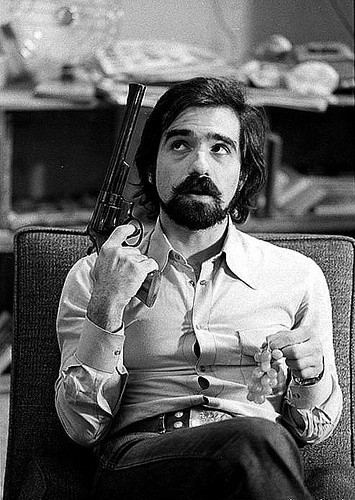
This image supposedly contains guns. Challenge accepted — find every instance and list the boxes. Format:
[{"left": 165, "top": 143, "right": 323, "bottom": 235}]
[{"left": 85, "top": 82, "right": 163, "bottom": 309}]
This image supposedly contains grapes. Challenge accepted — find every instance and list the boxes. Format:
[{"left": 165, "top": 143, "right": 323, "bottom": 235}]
[{"left": 246, "top": 346, "right": 283, "bottom": 404}]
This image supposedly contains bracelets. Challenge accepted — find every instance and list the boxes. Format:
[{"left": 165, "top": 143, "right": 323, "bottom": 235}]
[{"left": 292, "top": 370, "right": 325, "bottom": 387}]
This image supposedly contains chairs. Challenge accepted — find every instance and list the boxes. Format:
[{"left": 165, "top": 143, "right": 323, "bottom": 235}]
[{"left": 0, "top": 226, "right": 355, "bottom": 500}]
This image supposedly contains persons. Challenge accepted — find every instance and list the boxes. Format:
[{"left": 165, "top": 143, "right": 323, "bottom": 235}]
[{"left": 54, "top": 77, "right": 344, "bottom": 500}]
